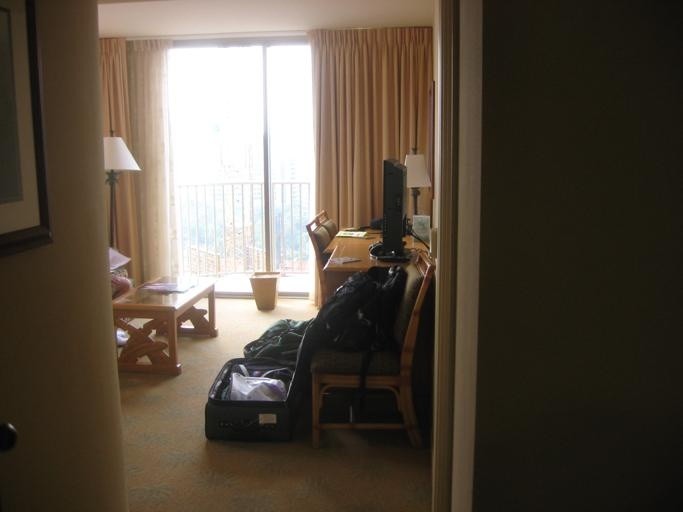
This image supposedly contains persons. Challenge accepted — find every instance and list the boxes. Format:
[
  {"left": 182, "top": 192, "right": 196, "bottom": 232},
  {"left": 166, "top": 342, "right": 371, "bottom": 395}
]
[{"left": 111, "top": 275, "right": 133, "bottom": 348}]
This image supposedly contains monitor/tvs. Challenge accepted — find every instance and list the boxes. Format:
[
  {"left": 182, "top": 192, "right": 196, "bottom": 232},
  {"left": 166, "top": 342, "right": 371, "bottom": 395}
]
[{"left": 370, "top": 160, "right": 417, "bottom": 261}]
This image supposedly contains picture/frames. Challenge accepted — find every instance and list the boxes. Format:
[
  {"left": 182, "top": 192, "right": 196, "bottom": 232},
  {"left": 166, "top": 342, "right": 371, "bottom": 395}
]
[{"left": 0, "top": 0, "right": 54, "bottom": 258}]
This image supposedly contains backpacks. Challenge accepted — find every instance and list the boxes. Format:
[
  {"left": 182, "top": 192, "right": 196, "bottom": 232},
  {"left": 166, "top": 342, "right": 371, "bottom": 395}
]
[{"left": 314, "top": 264, "right": 407, "bottom": 353}]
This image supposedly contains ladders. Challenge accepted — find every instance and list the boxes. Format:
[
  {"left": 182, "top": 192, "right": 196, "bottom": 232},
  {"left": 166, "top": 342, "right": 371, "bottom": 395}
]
[{"left": 250, "top": 272, "right": 281, "bottom": 310}]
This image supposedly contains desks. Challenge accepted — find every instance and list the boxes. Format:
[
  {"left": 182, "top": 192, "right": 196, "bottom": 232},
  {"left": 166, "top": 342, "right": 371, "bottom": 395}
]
[{"left": 320, "top": 227, "right": 430, "bottom": 307}]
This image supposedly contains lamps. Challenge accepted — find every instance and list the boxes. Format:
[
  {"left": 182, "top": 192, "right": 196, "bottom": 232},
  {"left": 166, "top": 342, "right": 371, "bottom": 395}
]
[
  {"left": 404, "top": 147, "right": 433, "bottom": 215},
  {"left": 102, "top": 136, "right": 144, "bottom": 253}
]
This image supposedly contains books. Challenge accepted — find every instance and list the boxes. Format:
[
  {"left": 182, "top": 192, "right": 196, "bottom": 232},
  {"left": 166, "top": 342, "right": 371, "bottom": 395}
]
[{"left": 336, "top": 230, "right": 368, "bottom": 239}]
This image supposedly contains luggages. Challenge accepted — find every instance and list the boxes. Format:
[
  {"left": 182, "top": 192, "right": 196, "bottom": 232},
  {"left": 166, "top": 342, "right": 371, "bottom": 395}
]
[{"left": 204, "top": 320, "right": 313, "bottom": 442}]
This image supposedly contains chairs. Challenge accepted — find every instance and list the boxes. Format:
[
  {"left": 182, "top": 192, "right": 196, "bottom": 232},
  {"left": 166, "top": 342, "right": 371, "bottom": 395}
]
[
  {"left": 304, "top": 208, "right": 340, "bottom": 269},
  {"left": 307, "top": 249, "right": 436, "bottom": 450},
  {"left": 110, "top": 276, "right": 132, "bottom": 301}
]
